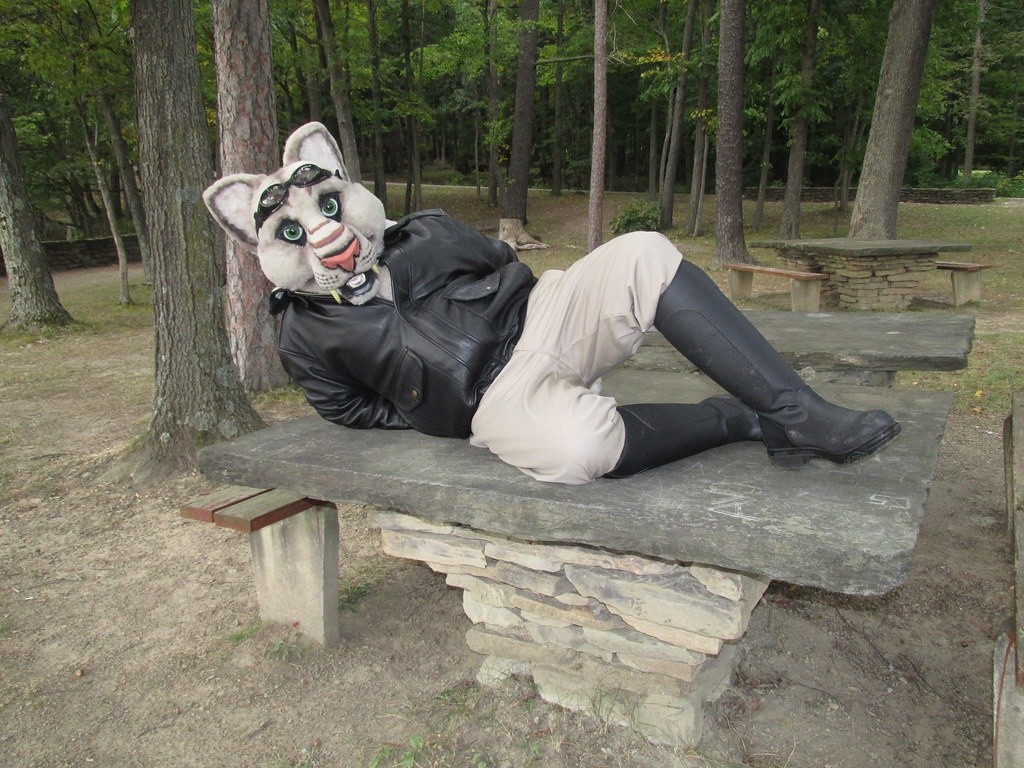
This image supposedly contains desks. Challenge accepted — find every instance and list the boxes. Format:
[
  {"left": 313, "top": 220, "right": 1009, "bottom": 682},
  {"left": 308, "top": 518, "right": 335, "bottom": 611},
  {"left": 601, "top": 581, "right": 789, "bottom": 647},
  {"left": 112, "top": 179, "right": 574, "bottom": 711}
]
[
  {"left": 749, "top": 236, "right": 972, "bottom": 309},
  {"left": 190, "top": 372, "right": 957, "bottom": 748},
  {"left": 634, "top": 313, "right": 976, "bottom": 389}
]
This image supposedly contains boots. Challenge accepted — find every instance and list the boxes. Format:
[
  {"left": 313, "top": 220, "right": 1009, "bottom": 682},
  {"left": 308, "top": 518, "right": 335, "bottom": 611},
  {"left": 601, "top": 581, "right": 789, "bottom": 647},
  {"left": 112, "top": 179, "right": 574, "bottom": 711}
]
[
  {"left": 653, "top": 259, "right": 902, "bottom": 469},
  {"left": 603, "top": 393, "right": 762, "bottom": 479}
]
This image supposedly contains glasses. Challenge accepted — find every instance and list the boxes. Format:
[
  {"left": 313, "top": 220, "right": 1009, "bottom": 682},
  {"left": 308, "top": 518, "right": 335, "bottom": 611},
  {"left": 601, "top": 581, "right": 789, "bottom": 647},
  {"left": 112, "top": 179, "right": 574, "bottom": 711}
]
[{"left": 257, "top": 163, "right": 328, "bottom": 221}]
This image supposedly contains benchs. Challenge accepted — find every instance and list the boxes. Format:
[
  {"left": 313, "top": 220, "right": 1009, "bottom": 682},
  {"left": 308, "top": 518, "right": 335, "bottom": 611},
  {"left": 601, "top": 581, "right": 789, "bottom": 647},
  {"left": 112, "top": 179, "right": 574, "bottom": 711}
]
[
  {"left": 935, "top": 261, "right": 993, "bottom": 309},
  {"left": 722, "top": 262, "right": 828, "bottom": 312},
  {"left": 180, "top": 486, "right": 341, "bottom": 650}
]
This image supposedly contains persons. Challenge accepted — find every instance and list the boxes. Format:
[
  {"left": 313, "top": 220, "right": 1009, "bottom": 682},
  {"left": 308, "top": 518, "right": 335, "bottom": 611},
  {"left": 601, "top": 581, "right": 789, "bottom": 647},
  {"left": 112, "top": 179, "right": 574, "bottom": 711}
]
[{"left": 203, "top": 121, "right": 897, "bottom": 488}]
